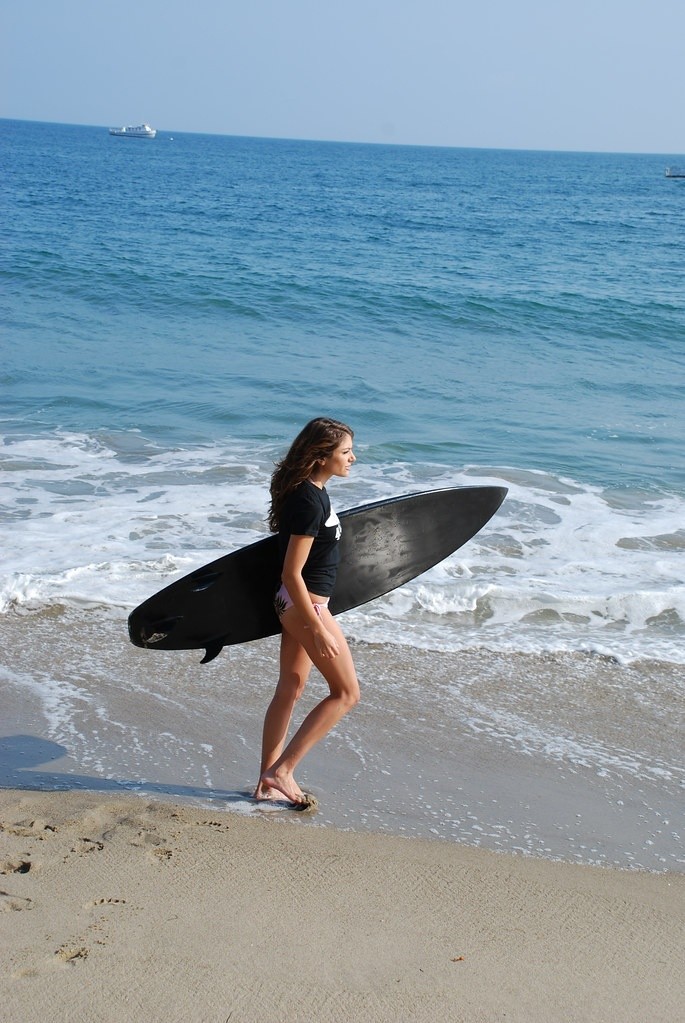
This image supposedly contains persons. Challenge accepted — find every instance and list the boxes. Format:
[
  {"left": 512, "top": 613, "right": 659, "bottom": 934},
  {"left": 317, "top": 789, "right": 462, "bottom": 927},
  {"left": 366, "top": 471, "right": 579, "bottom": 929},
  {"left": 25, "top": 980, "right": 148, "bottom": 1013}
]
[{"left": 254, "top": 417, "right": 361, "bottom": 805}]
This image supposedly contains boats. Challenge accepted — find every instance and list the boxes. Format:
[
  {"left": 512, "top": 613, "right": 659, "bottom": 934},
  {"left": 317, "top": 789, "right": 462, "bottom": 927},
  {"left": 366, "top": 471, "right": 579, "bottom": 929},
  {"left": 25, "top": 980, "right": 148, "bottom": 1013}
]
[{"left": 107, "top": 123, "right": 157, "bottom": 138}]
